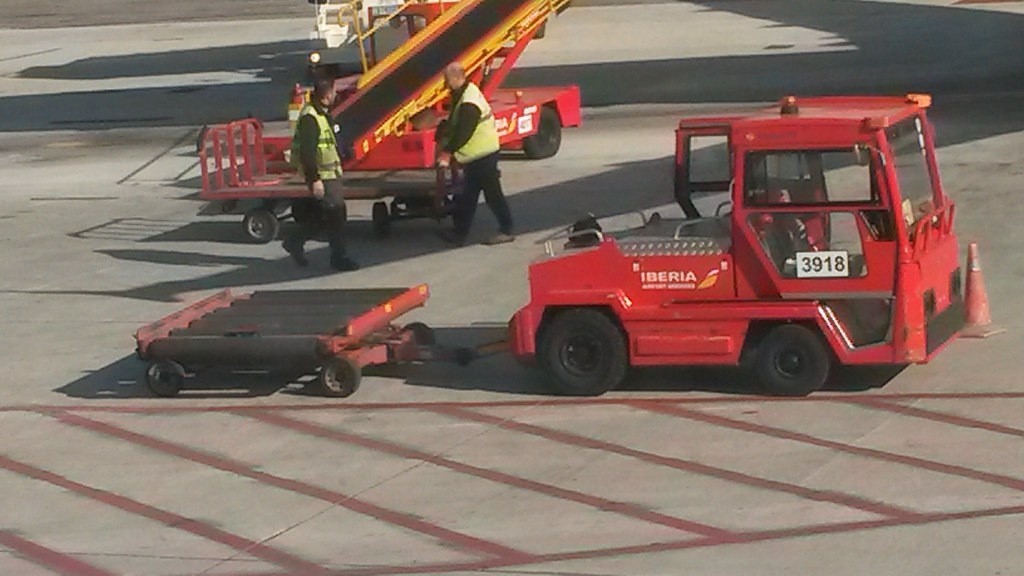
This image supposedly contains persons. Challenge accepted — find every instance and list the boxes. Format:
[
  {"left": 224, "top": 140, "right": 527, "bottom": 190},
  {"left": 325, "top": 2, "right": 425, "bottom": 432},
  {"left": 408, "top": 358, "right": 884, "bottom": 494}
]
[
  {"left": 435, "top": 62, "right": 518, "bottom": 246},
  {"left": 281, "top": 76, "right": 362, "bottom": 272}
]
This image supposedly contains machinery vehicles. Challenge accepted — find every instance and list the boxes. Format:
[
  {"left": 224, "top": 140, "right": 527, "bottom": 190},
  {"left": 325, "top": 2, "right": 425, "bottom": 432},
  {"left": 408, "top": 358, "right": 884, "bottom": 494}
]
[
  {"left": 250, "top": 0, "right": 580, "bottom": 172},
  {"left": 505, "top": 90, "right": 970, "bottom": 400}
]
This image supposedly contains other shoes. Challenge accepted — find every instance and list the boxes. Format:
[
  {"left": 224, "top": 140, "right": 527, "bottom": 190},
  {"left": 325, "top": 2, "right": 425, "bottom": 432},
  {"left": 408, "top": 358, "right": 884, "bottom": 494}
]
[
  {"left": 436, "top": 227, "right": 465, "bottom": 246},
  {"left": 330, "top": 260, "right": 360, "bottom": 271},
  {"left": 281, "top": 239, "right": 307, "bottom": 265},
  {"left": 482, "top": 232, "right": 516, "bottom": 244}
]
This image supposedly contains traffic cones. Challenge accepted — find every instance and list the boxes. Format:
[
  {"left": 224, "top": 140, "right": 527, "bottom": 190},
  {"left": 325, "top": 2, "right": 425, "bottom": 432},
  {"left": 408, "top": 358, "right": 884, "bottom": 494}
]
[{"left": 952, "top": 242, "right": 1010, "bottom": 339}]
